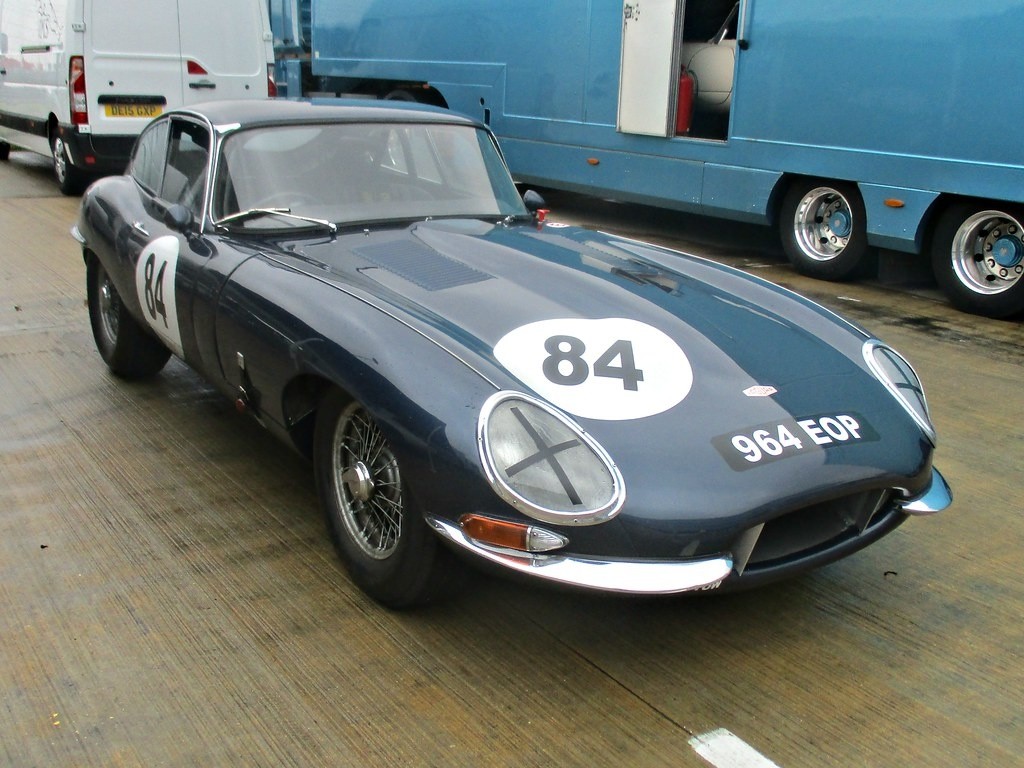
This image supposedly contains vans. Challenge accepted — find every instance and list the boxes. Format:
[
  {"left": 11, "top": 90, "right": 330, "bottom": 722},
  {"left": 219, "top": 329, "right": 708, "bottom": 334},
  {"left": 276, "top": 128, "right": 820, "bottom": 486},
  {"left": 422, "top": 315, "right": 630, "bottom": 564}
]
[{"left": 0, "top": 0, "right": 278, "bottom": 194}]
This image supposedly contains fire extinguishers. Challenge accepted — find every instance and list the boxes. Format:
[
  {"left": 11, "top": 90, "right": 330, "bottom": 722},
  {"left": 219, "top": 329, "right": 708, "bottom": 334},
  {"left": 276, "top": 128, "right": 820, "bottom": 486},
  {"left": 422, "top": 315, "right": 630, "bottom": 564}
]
[{"left": 676, "top": 64, "right": 698, "bottom": 133}]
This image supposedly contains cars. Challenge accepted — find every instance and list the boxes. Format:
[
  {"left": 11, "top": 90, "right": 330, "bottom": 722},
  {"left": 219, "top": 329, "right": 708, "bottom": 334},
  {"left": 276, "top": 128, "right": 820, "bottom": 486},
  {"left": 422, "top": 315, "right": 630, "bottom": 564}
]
[{"left": 70, "top": 97, "right": 955, "bottom": 612}]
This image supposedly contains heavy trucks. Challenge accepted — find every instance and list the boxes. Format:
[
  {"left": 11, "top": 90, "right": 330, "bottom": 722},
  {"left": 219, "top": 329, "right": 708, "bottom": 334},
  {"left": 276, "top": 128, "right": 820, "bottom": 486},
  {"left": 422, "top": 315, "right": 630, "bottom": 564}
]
[{"left": 263, "top": 0, "right": 1024, "bottom": 321}]
